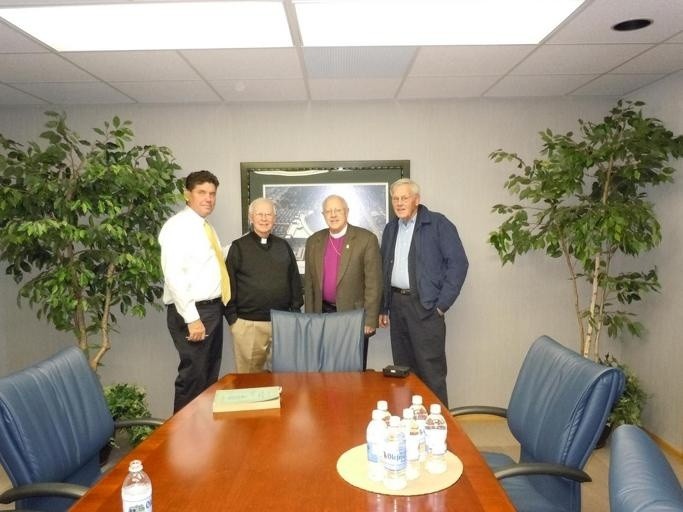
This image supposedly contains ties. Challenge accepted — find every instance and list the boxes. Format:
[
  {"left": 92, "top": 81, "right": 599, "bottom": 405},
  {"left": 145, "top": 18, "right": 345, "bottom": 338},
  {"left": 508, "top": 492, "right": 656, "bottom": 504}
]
[{"left": 205, "top": 223, "right": 232, "bottom": 307}]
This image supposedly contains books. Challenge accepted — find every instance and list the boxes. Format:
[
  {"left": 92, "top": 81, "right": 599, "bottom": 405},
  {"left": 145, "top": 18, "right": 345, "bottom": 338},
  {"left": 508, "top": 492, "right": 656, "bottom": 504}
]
[{"left": 212, "top": 386, "right": 282, "bottom": 413}]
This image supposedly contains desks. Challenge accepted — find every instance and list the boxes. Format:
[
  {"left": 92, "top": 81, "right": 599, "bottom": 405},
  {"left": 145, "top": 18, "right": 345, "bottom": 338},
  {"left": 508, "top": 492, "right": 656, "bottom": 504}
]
[{"left": 67, "top": 372, "right": 518, "bottom": 512}]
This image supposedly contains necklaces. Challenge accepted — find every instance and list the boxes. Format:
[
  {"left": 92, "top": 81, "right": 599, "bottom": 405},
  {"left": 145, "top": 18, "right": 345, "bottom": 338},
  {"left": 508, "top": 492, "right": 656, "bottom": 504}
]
[{"left": 327, "top": 234, "right": 340, "bottom": 256}]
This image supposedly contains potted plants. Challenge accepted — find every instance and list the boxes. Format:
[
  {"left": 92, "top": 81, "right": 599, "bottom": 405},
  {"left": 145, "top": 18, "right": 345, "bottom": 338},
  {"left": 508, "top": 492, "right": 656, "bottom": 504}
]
[
  {"left": 0, "top": 110, "right": 190, "bottom": 468},
  {"left": 486, "top": 99, "right": 683, "bottom": 449}
]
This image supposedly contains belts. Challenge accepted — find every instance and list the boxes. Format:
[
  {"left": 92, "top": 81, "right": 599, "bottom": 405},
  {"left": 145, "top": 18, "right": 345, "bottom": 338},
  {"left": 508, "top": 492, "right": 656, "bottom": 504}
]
[
  {"left": 197, "top": 298, "right": 221, "bottom": 306},
  {"left": 395, "top": 288, "right": 409, "bottom": 295}
]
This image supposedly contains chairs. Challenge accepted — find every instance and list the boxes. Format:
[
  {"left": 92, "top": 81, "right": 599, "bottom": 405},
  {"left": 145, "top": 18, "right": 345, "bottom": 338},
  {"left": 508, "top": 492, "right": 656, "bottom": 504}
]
[
  {"left": 0, "top": 346, "right": 166, "bottom": 512},
  {"left": 450, "top": 335, "right": 625, "bottom": 512},
  {"left": 270, "top": 308, "right": 365, "bottom": 372},
  {"left": 609, "top": 424, "right": 683, "bottom": 512}
]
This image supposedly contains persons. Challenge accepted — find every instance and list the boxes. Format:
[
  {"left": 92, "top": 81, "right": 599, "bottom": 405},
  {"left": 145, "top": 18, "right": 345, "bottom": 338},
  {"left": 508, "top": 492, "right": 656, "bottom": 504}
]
[
  {"left": 304, "top": 195, "right": 382, "bottom": 372},
  {"left": 157, "top": 170, "right": 233, "bottom": 416},
  {"left": 223, "top": 196, "right": 304, "bottom": 374},
  {"left": 378, "top": 177, "right": 469, "bottom": 410}
]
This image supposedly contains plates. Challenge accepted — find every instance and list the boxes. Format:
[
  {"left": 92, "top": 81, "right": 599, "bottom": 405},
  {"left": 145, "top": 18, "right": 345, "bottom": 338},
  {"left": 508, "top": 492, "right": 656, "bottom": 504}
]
[{"left": 336, "top": 442, "right": 464, "bottom": 497}]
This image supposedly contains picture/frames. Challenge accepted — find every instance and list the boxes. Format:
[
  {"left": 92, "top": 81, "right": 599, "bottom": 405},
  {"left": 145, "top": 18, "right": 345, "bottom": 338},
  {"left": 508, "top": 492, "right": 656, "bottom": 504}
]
[{"left": 241, "top": 160, "right": 410, "bottom": 294}]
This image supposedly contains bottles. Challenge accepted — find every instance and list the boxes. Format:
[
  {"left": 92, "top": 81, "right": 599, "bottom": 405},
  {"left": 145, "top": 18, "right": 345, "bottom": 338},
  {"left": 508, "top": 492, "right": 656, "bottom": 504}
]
[
  {"left": 121, "top": 459, "right": 153, "bottom": 512},
  {"left": 365, "top": 395, "right": 448, "bottom": 489}
]
